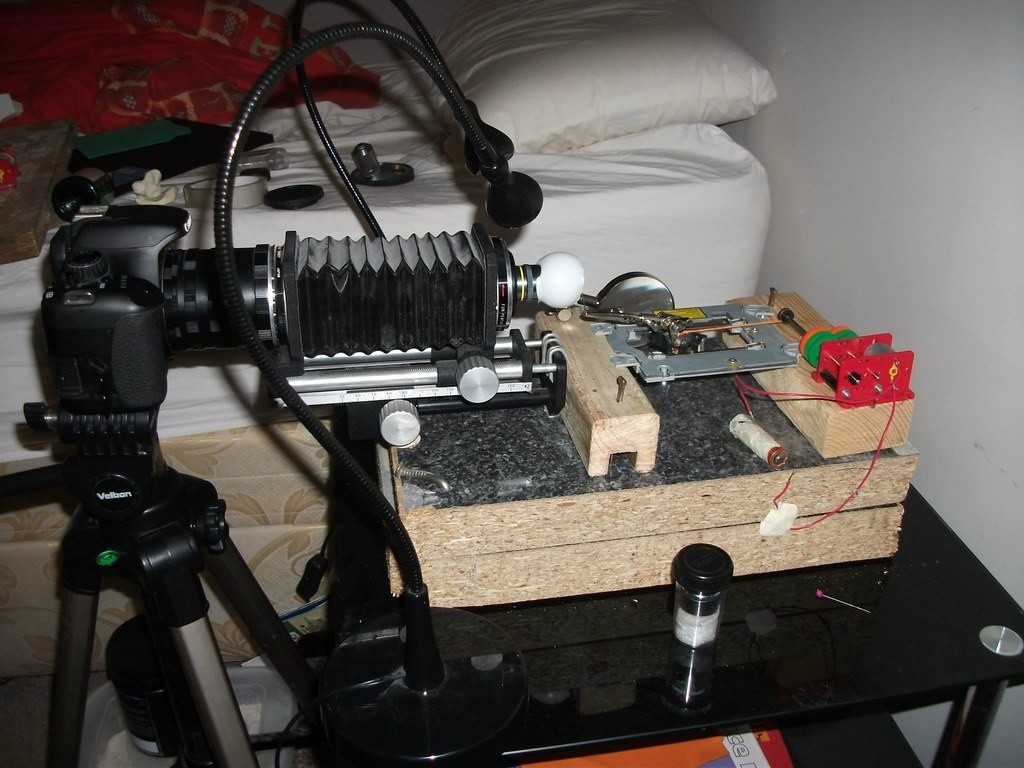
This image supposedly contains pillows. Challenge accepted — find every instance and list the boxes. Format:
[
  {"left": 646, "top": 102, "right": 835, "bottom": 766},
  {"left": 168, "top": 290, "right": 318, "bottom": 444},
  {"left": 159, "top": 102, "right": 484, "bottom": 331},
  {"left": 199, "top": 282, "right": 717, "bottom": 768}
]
[{"left": 423, "top": 0, "right": 777, "bottom": 165}]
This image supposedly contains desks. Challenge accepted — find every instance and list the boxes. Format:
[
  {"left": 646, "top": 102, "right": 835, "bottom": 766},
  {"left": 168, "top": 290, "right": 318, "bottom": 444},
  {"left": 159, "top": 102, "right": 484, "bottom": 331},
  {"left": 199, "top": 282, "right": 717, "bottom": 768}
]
[{"left": 457, "top": 485, "right": 1024, "bottom": 768}]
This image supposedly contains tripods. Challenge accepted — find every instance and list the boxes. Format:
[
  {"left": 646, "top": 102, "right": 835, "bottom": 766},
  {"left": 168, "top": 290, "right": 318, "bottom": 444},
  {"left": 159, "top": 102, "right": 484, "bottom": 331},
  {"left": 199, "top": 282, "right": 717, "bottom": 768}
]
[{"left": 22, "top": 401, "right": 325, "bottom": 768}]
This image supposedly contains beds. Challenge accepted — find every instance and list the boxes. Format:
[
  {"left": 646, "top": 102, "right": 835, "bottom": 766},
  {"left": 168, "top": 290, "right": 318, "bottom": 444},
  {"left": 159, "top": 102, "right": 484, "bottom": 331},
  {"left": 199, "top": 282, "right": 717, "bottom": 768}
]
[{"left": 0, "top": 57, "right": 770, "bottom": 678}]
